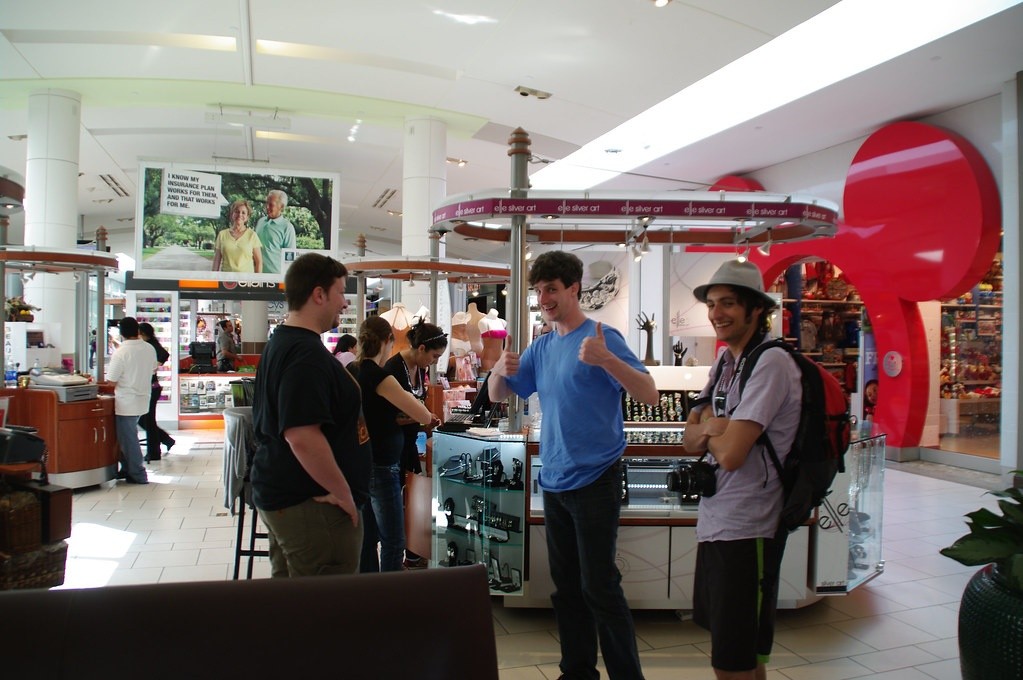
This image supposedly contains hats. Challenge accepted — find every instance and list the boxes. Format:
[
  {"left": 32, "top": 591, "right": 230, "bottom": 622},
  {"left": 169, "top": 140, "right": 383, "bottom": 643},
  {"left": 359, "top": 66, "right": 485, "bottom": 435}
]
[{"left": 691, "top": 260, "right": 776, "bottom": 312}]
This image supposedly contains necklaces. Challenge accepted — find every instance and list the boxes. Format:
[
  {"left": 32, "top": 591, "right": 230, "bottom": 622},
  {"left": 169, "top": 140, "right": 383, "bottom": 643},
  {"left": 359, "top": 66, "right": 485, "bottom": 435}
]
[{"left": 401, "top": 360, "right": 423, "bottom": 396}]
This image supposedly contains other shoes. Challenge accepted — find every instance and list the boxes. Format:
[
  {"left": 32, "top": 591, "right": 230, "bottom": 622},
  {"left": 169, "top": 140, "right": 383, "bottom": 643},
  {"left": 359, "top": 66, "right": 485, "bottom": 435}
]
[
  {"left": 115, "top": 470, "right": 125, "bottom": 479},
  {"left": 163, "top": 437, "right": 175, "bottom": 450},
  {"left": 125, "top": 477, "right": 148, "bottom": 484},
  {"left": 143, "top": 450, "right": 161, "bottom": 461},
  {"left": 403, "top": 556, "right": 428, "bottom": 569}
]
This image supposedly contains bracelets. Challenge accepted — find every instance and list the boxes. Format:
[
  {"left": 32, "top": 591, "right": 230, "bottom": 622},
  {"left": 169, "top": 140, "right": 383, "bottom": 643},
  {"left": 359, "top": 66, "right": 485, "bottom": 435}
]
[{"left": 703, "top": 417, "right": 708, "bottom": 422}]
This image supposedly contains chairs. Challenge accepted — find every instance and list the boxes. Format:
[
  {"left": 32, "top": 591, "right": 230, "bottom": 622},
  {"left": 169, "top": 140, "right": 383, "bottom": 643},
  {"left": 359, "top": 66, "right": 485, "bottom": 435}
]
[{"left": 223, "top": 406, "right": 268, "bottom": 579}]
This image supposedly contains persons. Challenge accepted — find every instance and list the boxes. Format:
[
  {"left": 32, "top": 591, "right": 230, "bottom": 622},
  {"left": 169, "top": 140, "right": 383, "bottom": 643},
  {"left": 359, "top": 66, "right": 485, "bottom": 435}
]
[
  {"left": 378, "top": 302, "right": 417, "bottom": 357},
  {"left": 864, "top": 378, "right": 879, "bottom": 420},
  {"left": 216, "top": 320, "right": 243, "bottom": 372},
  {"left": 378, "top": 317, "right": 449, "bottom": 569},
  {"left": 105, "top": 317, "right": 157, "bottom": 484},
  {"left": 138, "top": 320, "right": 175, "bottom": 462},
  {"left": 344, "top": 316, "right": 431, "bottom": 575},
  {"left": 487, "top": 251, "right": 658, "bottom": 680},
  {"left": 681, "top": 256, "right": 801, "bottom": 680},
  {"left": 210, "top": 201, "right": 263, "bottom": 273},
  {"left": 464, "top": 302, "right": 486, "bottom": 354},
  {"left": 88, "top": 330, "right": 97, "bottom": 369},
  {"left": 451, "top": 311, "right": 474, "bottom": 359},
  {"left": 254, "top": 191, "right": 296, "bottom": 275},
  {"left": 251, "top": 254, "right": 372, "bottom": 579},
  {"left": 332, "top": 334, "right": 358, "bottom": 368},
  {"left": 477, "top": 308, "right": 506, "bottom": 372}
]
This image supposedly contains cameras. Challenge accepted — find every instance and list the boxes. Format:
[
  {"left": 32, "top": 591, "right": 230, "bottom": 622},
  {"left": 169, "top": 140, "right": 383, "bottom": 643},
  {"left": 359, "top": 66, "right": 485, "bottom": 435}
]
[{"left": 667, "top": 459, "right": 717, "bottom": 498}]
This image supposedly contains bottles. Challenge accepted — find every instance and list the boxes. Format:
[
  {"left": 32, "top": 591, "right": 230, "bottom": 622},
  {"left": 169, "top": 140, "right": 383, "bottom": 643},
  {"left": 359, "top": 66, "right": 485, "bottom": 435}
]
[
  {"left": 5, "top": 361, "right": 17, "bottom": 389},
  {"left": 33, "top": 359, "right": 42, "bottom": 375}
]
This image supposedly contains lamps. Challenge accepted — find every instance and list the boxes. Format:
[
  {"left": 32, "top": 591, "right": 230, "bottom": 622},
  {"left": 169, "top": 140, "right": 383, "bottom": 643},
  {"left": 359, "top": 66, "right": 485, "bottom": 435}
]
[
  {"left": 408, "top": 273, "right": 414, "bottom": 287},
  {"left": 641, "top": 225, "right": 650, "bottom": 253},
  {"left": 738, "top": 238, "right": 750, "bottom": 262},
  {"left": 757, "top": 228, "right": 773, "bottom": 256},
  {"left": 378, "top": 274, "right": 383, "bottom": 290},
  {"left": 631, "top": 236, "right": 642, "bottom": 261}
]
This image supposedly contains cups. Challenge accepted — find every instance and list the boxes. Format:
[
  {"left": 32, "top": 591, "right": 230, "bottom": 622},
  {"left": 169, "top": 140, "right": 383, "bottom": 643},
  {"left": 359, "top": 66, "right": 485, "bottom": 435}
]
[{"left": 18, "top": 375, "right": 29, "bottom": 388}]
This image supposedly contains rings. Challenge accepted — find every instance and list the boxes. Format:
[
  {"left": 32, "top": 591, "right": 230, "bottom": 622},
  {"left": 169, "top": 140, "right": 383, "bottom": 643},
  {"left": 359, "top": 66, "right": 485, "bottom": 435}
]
[{"left": 512, "top": 359, "right": 514, "bottom": 364}]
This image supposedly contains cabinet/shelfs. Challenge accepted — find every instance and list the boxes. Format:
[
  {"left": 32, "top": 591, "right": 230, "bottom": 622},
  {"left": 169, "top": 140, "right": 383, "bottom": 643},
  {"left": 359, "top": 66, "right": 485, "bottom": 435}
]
[{"left": 57, "top": 395, "right": 117, "bottom": 488}]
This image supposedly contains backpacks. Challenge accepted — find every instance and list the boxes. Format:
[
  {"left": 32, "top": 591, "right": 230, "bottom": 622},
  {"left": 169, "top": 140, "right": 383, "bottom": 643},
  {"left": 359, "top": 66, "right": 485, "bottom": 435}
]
[{"left": 707, "top": 338, "right": 853, "bottom": 531}]
